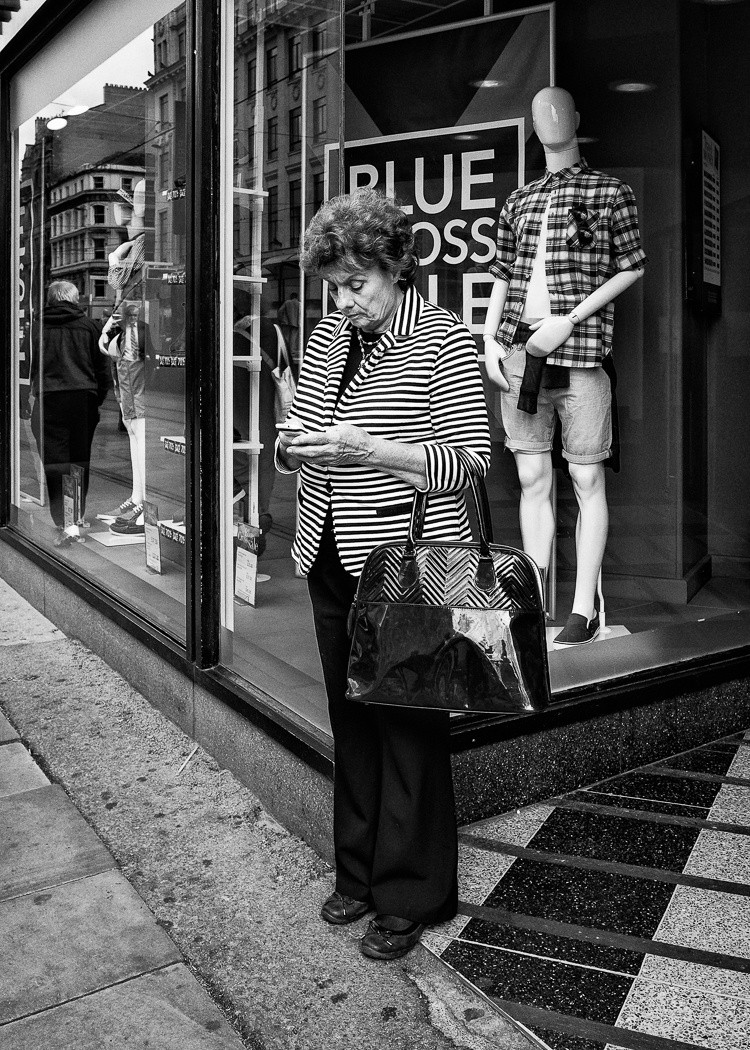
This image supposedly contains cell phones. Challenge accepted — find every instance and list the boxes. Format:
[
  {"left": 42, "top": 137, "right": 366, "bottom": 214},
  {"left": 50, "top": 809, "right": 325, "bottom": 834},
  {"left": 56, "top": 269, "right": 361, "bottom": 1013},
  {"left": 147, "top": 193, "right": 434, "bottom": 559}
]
[{"left": 276, "top": 422, "right": 310, "bottom": 437}]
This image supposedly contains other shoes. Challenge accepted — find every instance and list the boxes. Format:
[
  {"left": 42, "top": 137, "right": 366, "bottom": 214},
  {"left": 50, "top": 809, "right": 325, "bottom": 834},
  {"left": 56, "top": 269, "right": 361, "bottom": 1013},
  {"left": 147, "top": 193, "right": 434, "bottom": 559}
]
[
  {"left": 554, "top": 607, "right": 601, "bottom": 645},
  {"left": 74, "top": 517, "right": 91, "bottom": 530},
  {"left": 53, "top": 531, "right": 71, "bottom": 547},
  {"left": 257, "top": 513, "right": 273, "bottom": 558},
  {"left": 109, "top": 510, "right": 145, "bottom": 536},
  {"left": 107, "top": 497, "right": 138, "bottom": 517},
  {"left": 321, "top": 890, "right": 373, "bottom": 925},
  {"left": 361, "top": 915, "right": 426, "bottom": 961},
  {"left": 120, "top": 503, "right": 144, "bottom": 519}
]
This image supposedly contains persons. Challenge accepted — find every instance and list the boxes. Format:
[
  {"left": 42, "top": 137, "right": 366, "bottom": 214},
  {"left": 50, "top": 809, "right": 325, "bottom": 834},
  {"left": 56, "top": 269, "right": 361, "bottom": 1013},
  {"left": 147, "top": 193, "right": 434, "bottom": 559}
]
[
  {"left": 234, "top": 287, "right": 301, "bottom": 534},
  {"left": 107, "top": 178, "right": 146, "bottom": 536},
  {"left": 274, "top": 188, "right": 492, "bottom": 961},
  {"left": 483, "top": 88, "right": 646, "bottom": 647},
  {"left": 24, "top": 282, "right": 156, "bottom": 544}
]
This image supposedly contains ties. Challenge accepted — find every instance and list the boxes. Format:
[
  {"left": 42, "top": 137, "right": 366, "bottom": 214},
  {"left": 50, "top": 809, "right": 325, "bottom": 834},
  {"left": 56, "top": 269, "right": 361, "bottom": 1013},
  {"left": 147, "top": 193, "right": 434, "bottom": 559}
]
[{"left": 130, "top": 325, "right": 140, "bottom": 361}]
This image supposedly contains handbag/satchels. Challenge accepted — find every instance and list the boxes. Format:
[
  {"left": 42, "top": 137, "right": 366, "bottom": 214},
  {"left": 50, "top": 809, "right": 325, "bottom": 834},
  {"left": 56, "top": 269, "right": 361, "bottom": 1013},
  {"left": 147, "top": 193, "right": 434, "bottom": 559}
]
[
  {"left": 271, "top": 324, "right": 296, "bottom": 424},
  {"left": 345, "top": 448, "right": 553, "bottom": 715}
]
